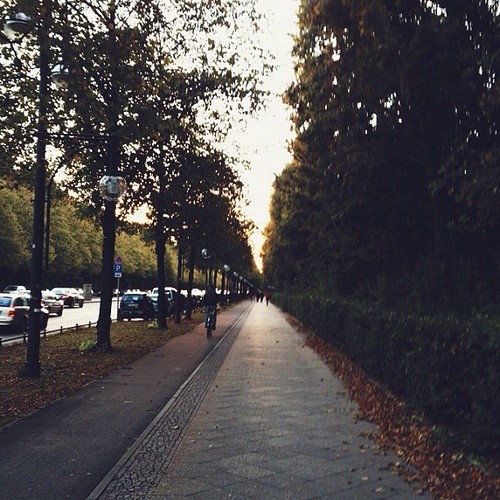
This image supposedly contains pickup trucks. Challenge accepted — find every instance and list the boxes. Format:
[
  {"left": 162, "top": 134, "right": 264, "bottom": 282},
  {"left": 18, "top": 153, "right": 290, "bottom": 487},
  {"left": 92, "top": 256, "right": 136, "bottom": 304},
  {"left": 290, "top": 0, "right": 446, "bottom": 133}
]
[{"left": 150, "top": 286, "right": 177, "bottom": 315}]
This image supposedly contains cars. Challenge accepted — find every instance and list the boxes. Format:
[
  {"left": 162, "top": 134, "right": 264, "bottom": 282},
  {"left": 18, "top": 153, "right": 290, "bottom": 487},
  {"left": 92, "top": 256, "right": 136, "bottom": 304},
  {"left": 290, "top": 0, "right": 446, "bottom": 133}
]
[
  {"left": 118, "top": 292, "right": 155, "bottom": 321},
  {"left": 122, "top": 289, "right": 151, "bottom": 296},
  {"left": 0, "top": 285, "right": 84, "bottom": 332},
  {"left": 113, "top": 289, "right": 119, "bottom": 296}
]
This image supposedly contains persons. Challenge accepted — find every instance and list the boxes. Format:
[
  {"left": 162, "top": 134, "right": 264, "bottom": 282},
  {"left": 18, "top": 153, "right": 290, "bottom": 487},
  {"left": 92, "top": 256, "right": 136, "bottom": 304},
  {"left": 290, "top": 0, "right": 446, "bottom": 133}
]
[
  {"left": 248, "top": 287, "right": 271, "bottom": 306},
  {"left": 203, "top": 284, "right": 217, "bottom": 330},
  {"left": 143, "top": 291, "right": 202, "bottom": 321}
]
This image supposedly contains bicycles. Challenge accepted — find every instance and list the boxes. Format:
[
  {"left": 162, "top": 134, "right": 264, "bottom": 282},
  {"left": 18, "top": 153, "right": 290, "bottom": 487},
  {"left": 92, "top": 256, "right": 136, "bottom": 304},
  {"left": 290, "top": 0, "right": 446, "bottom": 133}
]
[{"left": 205, "top": 308, "right": 213, "bottom": 338}]
